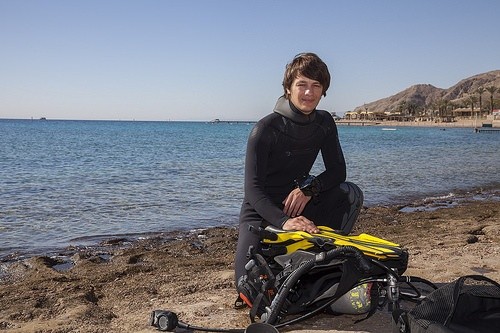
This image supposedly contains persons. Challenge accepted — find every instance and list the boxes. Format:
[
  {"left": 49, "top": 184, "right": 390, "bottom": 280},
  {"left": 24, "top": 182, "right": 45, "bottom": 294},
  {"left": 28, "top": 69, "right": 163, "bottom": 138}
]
[{"left": 231, "top": 52, "right": 364, "bottom": 309}]
